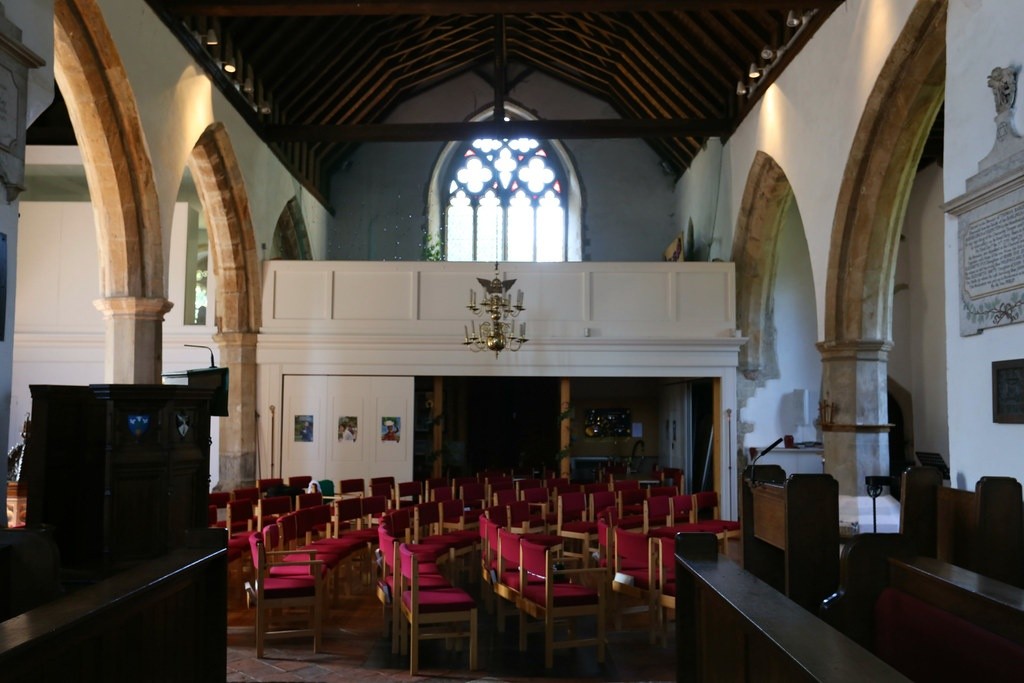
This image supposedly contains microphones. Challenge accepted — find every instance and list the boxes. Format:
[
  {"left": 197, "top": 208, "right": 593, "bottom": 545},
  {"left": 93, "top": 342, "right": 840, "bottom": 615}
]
[
  {"left": 752, "top": 438, "right": 783, "bottom": 485},
  {"left": 184, "top": 345, "right": 217, "bottom": 368}
]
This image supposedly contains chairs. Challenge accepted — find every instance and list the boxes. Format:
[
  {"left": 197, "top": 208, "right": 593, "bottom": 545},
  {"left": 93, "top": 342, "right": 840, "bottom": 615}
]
[{"left": 208, "top": 460, "right": 741, "bottom": 675}]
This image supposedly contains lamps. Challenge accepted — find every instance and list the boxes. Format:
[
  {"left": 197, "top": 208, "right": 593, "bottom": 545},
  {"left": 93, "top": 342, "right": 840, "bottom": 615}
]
[{"left": 458, "top": 15, "right": 528, "bottom": 359}]
